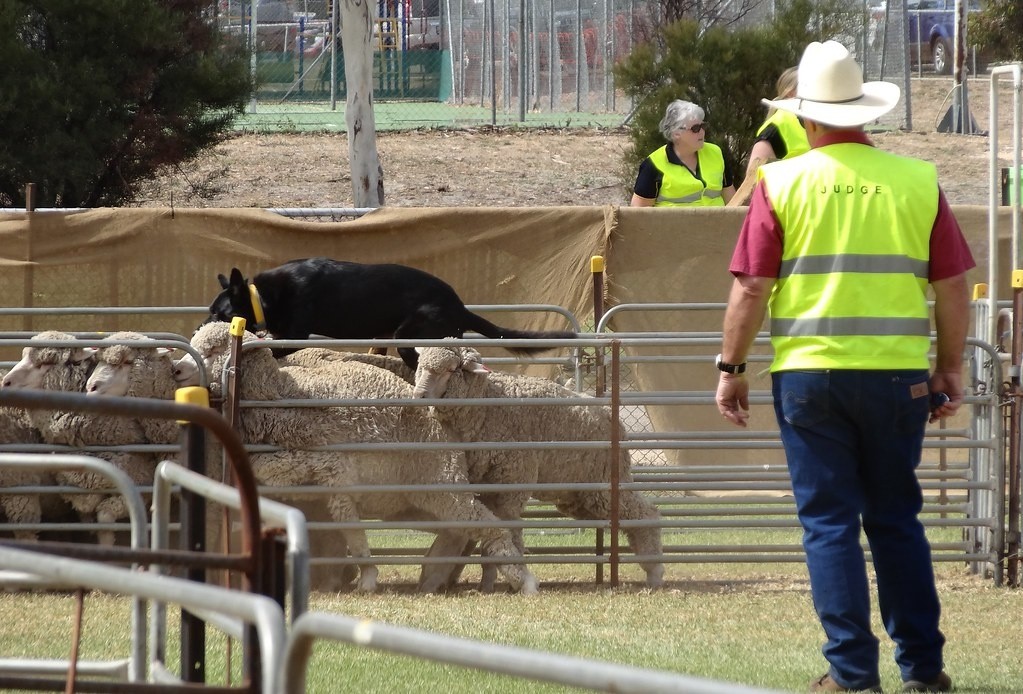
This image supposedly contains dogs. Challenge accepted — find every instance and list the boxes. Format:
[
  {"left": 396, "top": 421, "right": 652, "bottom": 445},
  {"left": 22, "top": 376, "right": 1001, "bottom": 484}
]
[{"left": 193, "top": 257, "right": 578, "bottom": 376}]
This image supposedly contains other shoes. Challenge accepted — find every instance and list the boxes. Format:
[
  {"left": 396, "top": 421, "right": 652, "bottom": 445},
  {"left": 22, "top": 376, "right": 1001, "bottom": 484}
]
[
  {"left": 900, "top": 667, "right": 951, "bottom": 694},
  {"left": 812, "top": 672, "right": 884, "bottom": 694}
]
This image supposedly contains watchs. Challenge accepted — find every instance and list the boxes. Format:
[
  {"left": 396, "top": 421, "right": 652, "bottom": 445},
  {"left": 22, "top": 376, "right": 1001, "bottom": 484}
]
[{"left": 716, "top": 353, "right": 748, "bottom": 374}]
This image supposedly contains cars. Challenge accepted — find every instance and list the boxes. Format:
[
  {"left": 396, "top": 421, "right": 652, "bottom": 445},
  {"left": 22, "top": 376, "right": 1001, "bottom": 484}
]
[{"left": 872, "top": 0, "right": 993, "bottom": 77}]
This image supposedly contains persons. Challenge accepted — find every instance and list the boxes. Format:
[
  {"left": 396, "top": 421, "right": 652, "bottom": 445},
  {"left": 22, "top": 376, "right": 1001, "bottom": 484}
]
[
  {"left": 744, "top": 67, "right": 814, "bottom": 179},
  {"left": 713, "top": 40, "right": 981, "bottom": 694},
  {"left": 628, "top": 97, "right": 737, "bottom": 211}
]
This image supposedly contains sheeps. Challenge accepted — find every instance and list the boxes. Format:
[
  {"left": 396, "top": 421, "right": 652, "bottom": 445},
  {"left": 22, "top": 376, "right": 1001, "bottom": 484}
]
[{"left": 1, "top": 321, "right": 663, "bottom": 596}]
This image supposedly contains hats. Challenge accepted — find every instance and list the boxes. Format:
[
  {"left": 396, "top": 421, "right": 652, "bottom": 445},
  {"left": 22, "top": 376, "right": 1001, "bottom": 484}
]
[{"left": 761, "top": 40, "right": 901, "bottom": 128}]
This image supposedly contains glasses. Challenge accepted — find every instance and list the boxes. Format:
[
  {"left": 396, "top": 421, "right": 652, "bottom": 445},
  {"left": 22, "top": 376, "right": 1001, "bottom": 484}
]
[
  {"left": 797, "top": 116, "right": 805, "bottom": 129},
  {"left": 678, "top": 122, "right": 709, "bottom": 134}
]
